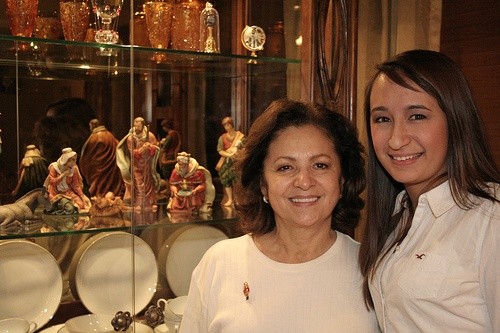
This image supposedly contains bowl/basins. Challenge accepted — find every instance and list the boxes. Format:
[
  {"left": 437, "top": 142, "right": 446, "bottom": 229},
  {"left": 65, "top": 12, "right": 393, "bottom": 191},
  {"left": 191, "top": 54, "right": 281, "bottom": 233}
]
[{"left": 65, "top": 314, "right": 120, "bottom": 333}]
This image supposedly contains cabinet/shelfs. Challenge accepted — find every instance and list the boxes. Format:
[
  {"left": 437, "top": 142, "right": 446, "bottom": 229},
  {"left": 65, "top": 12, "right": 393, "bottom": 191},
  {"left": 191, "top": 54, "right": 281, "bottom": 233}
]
[{"left": 0, "top": 0, "right": 302, "bottom": 238}]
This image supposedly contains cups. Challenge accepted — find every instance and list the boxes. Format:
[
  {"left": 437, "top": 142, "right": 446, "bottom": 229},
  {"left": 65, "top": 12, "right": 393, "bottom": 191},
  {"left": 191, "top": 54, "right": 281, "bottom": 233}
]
[
  {"left": 157, "top": 295, "right": 187, "bottom": 331},
  {"left": 6, "top": 0, "right": 124, "bottom": 62},
  {"left": 0, "top": 317, "right": 37, "bottom": 333},
  {"left": 143, "top": 0, "right": 203, "bottom": 64}
]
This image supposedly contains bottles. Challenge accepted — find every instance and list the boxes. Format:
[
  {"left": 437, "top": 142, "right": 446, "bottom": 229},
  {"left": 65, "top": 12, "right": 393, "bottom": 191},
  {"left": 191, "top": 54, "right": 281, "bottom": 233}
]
[{"left": 200, "top": 1, "right": 221, "bottom": 55}]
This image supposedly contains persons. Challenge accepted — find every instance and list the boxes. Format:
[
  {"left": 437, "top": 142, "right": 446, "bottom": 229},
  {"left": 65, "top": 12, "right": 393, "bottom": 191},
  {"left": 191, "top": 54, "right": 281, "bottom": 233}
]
[
  {"left": 10, "top": 116, "right": 244, "bottom": 212},
  {"left": 179, "top": 99, "right": 384, "bottom": 333},
  {"left": 357, "top": 50, "right": 500, "bottom": 333},
  {"left": 40, "top": 201, "right": 232, "bottom": 233}
]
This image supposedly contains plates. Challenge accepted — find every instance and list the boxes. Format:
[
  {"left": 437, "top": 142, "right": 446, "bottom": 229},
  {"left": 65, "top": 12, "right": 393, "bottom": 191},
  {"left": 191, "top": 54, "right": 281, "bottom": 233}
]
[
  {"left": 165, "top": 227, "right": 229, "bottom": 298},
  {"left": 154, "top": 323, "right": 172, "bottom": 333},
  {"left": 57, "top": 326, "right": 68, "bottom": 333},
  {"left": 75, "top": 232, "right": 158, "bottom": 318},
  {"left": 158, "top": 225, "right": 213, "bottom": 299},
  {"left": 0, "top": 241, "right": 64, "bottom": 333},
  {"left": 69, "top": 231, "right": 112, "bottom": 306}
]
